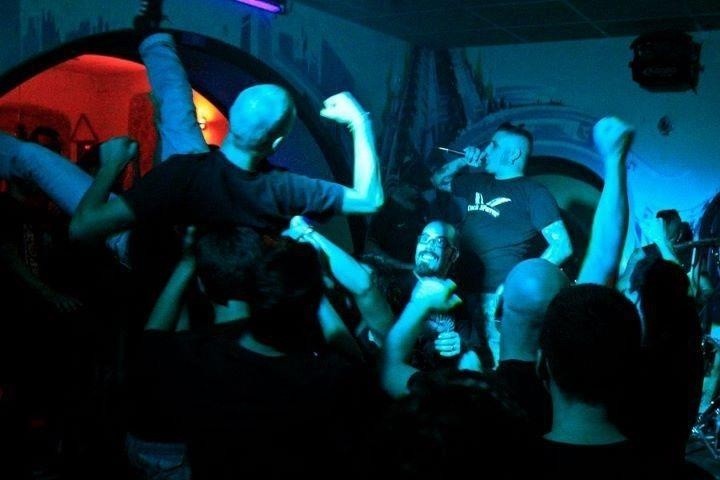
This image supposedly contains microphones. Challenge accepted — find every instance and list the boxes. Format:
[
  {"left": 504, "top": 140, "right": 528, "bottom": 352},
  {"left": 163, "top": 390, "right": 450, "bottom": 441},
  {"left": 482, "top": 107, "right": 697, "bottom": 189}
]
[{"left": 437, "top": 145, "right": 487, "bottom": 162}]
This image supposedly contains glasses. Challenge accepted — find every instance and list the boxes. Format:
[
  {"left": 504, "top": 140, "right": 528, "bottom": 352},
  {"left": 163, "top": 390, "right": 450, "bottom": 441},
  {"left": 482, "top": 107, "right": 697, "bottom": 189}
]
[{"left": 417, "top": 235, "right": 455, "bottom": 250}]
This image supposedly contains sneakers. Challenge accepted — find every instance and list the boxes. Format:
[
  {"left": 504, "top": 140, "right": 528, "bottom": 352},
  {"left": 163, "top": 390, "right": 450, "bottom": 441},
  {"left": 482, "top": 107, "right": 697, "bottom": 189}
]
[{"left": 133, "top": 0, "right": 163, "bottom": 32}]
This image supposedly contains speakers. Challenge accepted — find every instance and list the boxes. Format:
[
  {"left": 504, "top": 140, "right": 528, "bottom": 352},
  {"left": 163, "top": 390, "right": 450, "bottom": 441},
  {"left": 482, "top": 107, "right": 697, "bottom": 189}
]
[{"left": 628, "top": 38, "right": 700, "bottom": 86}]
